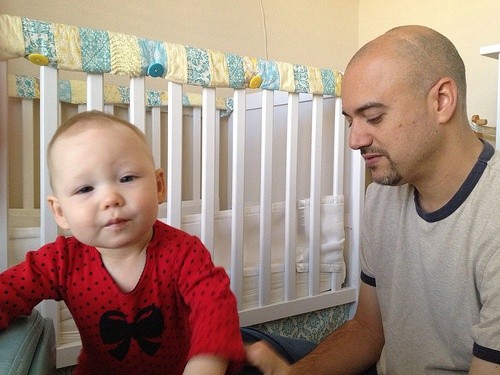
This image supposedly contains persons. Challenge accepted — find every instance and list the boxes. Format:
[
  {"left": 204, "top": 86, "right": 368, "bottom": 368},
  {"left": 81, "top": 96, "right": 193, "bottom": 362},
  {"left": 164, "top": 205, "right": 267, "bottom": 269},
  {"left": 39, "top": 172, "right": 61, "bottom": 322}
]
[
  {"left": 227, "top": 23, "right": 500, "bottom": 374},
  {"left": 1, "top": 108, "right": 249, "bottom": 375}
]
